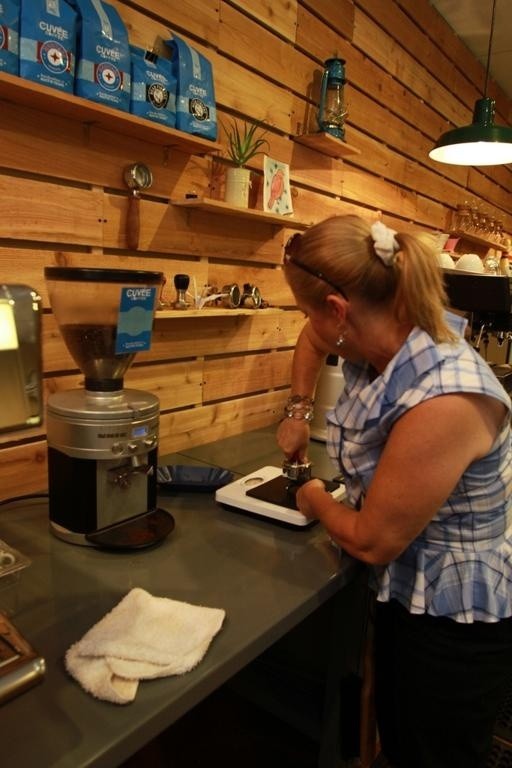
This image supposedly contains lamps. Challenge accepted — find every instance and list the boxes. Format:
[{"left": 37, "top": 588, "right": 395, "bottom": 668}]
[{"left": 428, "top": 0, "right": 512, "bottom": 170}]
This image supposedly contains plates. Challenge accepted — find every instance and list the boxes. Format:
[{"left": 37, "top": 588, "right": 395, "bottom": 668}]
[{"left": 158, "top": 461, "right": 231, "bottom": 491}]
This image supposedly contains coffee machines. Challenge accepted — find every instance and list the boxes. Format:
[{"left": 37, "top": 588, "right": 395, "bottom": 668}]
[{"left": 38, "top": 264, "right": 178, "bottom": 549}]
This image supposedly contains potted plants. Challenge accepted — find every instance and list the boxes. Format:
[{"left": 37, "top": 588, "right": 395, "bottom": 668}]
[{"left": 215, "top": 105, "right": 277, "bottom": 209}]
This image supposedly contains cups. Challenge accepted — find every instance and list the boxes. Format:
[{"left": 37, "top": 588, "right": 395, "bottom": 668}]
[
  {"left": 483, "top": 257, "right": 498, "bottom": 274},
  {"left": 453, "top": 205, "right": 504, "bottom": 242}
]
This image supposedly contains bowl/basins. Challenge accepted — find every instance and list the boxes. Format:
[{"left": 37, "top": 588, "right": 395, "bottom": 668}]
[{"left": 439, "top": 252, "right": 483, "bottom": 272}]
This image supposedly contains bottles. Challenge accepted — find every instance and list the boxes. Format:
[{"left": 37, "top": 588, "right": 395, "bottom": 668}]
[{"left": 500, "top": 253, "right": 508, "bottom": 275}]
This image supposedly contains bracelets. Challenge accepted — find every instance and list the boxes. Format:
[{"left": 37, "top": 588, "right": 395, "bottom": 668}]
[{"left": 282, "top": 396, "right": 315, "bottom": 421}]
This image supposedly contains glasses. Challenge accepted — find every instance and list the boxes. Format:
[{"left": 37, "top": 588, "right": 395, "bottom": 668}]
[
  {"left": 284, "top": 233, "right": 349, "bottom": 303},
  {"left": 196, "top": 284, "right": 261, "bottom": 308}
]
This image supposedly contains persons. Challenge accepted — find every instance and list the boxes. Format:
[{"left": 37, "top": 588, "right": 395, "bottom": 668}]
[{"left": 275, "top": 213, "right": 512, "bottom": 768}]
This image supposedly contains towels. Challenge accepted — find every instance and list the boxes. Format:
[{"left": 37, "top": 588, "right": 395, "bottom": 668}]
[{"left": 60, "top": 581, "right": 226, "bottom": 709}]
